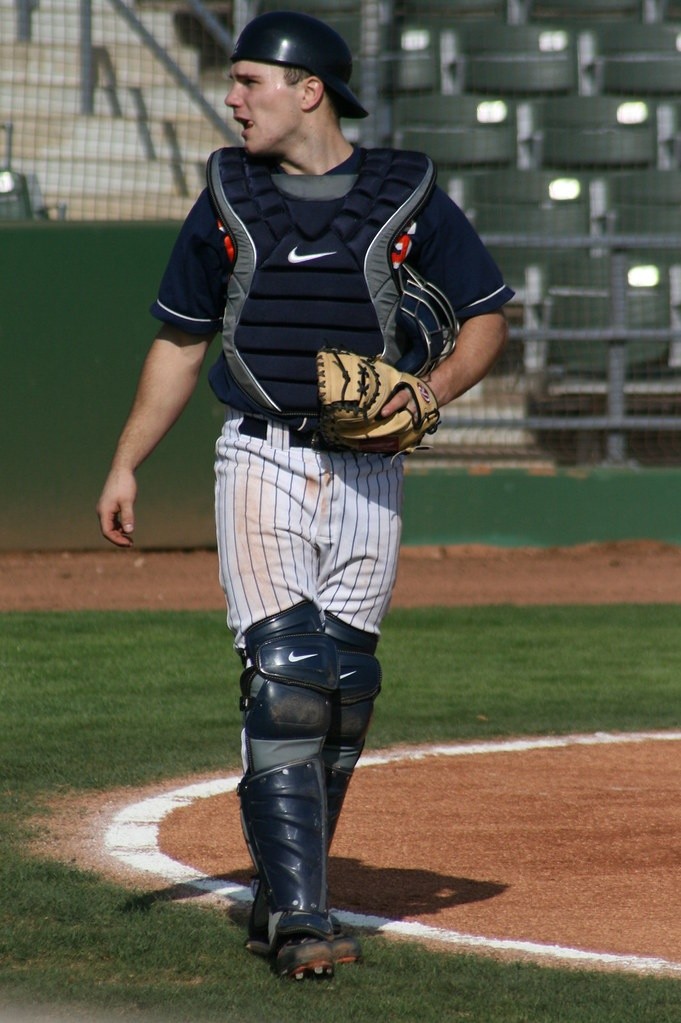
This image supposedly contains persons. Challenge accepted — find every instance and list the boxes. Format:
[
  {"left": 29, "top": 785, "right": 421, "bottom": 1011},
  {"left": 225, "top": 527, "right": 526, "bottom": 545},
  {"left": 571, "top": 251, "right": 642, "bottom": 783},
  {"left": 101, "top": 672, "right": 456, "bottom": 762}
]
[{"left": 96, "top": 14, "right": 514, "bottom": 980}]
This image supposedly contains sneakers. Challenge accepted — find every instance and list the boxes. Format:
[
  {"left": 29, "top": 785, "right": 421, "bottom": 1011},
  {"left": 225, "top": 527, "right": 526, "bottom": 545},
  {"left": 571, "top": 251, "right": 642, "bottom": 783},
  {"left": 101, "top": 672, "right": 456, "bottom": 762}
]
[
  {"left": 328, "top": 927, "right": 359, "bottom": 963},
  {"left": 276, "top": 934, "right": 336, "bottom": 980}
]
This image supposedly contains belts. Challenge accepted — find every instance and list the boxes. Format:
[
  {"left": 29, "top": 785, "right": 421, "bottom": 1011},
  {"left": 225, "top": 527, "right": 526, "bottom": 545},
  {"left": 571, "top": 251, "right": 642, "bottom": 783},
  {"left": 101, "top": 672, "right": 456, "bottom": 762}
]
[{"left": 239, "top": 415, "right": 335, "bottom": 451}]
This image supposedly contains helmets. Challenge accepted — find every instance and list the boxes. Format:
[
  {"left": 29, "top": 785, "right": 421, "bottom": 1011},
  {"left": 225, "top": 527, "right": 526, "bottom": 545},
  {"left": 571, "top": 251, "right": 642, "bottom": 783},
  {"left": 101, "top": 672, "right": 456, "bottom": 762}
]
[{"left": 231, "top": 11, "right": 370, "bottom": 119}]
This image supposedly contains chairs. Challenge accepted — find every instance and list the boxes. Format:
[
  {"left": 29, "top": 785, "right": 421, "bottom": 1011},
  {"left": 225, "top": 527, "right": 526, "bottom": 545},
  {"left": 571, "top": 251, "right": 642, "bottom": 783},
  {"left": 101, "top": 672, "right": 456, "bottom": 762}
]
[{"left": 173, "top": 0, "right": 681, "bottom": 466}]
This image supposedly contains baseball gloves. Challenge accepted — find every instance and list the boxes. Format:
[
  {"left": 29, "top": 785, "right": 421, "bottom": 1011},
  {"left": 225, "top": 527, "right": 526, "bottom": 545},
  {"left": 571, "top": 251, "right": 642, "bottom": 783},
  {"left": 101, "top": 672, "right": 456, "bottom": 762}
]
[{"left": 315, "top": 346, "right": 442, "bottom": 456}]
